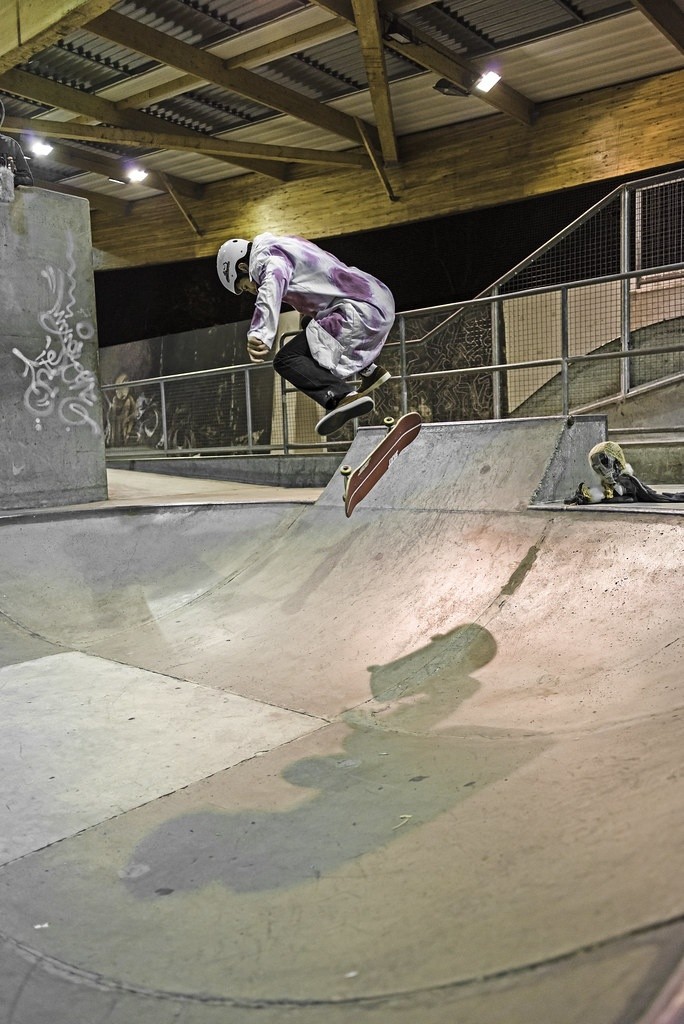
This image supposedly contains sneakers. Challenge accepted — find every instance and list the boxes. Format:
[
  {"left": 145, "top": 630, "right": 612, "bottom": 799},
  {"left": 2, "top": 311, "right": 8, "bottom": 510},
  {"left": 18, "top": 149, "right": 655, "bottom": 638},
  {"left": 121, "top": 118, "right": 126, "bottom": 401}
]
[
  {"left": 354, "top": 366, "right": 392, "bottom": 396},
  {"left": 315, "top": 393, "right": 375, "bottom": 438}
]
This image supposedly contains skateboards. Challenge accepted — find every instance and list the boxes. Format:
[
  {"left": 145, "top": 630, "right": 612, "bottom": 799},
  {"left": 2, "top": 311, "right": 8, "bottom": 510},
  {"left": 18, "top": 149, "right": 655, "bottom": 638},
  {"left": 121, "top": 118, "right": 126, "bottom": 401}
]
[{"left": 341, "top": 411, "right": 421, "bottom": 518}]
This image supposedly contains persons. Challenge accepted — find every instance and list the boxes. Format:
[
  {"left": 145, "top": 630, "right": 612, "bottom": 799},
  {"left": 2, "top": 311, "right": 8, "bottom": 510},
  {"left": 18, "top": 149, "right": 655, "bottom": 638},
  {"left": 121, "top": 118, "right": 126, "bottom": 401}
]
[{"left": 214, "top": 230, "right": 397, "bottom": 435}]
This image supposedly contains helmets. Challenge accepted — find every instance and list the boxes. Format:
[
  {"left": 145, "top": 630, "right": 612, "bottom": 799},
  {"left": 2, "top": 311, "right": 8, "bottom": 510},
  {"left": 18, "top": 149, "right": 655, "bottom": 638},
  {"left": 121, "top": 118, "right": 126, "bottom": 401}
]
[{"left": 215, "top": 238, "right": 251, "bottom": 296}]
